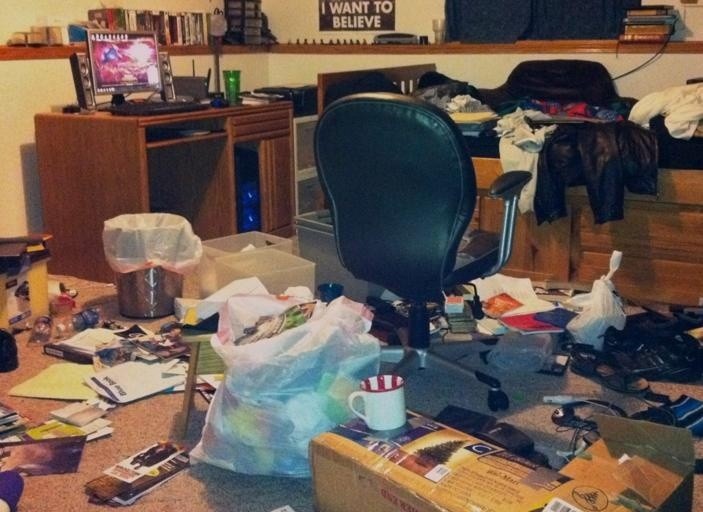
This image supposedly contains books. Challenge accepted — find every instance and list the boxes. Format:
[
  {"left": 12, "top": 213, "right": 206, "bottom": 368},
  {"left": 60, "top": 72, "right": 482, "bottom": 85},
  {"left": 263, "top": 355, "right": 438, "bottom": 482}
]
[
  {"left": 44, "top": 325, "right": 122, "bottom": 364},
  {"left": 0, "top": 404, "right": 21, "bottom": 426},
  {"left": 619, "top": 4, "right": 685, "bottom": 42},
  {"left": 88, "top": 7, "right": 211, "bottom": 47},
  {"left": 500, "top": 308, "right": 579, "bottom": 335}
]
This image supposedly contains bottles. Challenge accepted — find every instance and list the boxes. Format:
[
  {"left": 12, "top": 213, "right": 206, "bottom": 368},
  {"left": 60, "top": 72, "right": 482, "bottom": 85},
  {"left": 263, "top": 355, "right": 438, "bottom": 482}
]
[
  {"left": 56, "top": 303, "right": 104, "bottom": 334},
  {"left": 33, "top": 313, "right": 53, "bottom": 342}
]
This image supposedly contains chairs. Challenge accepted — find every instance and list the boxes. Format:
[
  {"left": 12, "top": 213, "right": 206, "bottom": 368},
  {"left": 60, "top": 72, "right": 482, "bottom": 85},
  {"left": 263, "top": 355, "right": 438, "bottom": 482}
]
[{"left": 313, "top": 92, "right": 533, "bottom": 412}]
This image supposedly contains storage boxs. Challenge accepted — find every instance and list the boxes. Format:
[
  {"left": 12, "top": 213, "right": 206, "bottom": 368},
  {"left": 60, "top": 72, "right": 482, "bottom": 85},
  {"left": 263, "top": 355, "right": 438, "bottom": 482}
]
[
  {"left": 195, "top": 209, "right": 385, "bottom": 303},
  {"left": 555, "top": 411, "right": 694, "bottom": 512},
  {"left": 0, "top": 242, "right": 50, "bottom": 335}
]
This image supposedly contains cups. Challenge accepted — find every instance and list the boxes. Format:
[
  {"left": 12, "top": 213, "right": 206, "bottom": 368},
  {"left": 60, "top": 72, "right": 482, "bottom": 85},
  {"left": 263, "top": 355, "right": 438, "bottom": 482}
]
[
  {"left": 432, "top": 19, "right": 445, "bottom": 43},
  {"left": 7, "top": 26, "right": 64, "bottom": 48},
  {"left": 223, "top": 69, "right": 241, "bottom": 106},
  {"left": 349, "top": 374, "right": 407, "bottom": 431}
]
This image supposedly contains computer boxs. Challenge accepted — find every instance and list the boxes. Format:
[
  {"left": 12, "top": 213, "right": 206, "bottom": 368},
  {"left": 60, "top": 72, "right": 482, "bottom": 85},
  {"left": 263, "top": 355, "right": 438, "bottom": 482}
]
[{"left": 233, "top": 143, "right": 261, "bottom": 233}]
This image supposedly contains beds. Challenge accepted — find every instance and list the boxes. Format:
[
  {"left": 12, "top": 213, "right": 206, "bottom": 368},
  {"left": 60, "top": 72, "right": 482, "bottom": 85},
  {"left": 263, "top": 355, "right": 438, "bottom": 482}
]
[{"left": 318, "top": 63, "right": 703, "bottom": 307}]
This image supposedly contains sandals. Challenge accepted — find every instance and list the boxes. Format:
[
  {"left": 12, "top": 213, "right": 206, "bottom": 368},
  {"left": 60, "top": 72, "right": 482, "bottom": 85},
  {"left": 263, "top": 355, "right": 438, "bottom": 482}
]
[{"left": 571, "top": 350, "right": 649, "bottom": 393}]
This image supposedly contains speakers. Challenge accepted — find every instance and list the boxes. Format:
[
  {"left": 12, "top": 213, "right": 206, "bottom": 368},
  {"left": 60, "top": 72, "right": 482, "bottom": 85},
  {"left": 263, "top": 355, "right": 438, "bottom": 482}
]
[
  {"left": 68, "top": 52, "right": 93, "bottom": 110},
  {"left": 158, "top": 51, "right": 176, "bottom": 102}
]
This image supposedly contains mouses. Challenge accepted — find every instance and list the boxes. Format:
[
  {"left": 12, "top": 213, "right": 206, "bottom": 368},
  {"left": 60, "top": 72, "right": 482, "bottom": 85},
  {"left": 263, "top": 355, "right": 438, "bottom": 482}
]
[{"left": 211, "top": 97, "right": 229, "bottom": 107}]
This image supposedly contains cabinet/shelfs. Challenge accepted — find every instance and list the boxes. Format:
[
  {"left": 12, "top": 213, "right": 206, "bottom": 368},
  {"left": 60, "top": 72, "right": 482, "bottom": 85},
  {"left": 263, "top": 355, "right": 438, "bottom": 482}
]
[{"left": 34, "top": 102, "right": 295, "bottom": 284}]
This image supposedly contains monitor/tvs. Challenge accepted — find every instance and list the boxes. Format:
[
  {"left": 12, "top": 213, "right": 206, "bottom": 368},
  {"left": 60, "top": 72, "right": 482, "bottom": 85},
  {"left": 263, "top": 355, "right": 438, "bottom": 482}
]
[{"left": 85, "top": 29, "right": 162, "bottom": 111}]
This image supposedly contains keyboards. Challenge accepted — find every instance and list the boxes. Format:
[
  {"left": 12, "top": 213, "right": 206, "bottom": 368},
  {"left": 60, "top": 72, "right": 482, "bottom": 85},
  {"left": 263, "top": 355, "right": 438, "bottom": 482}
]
[{"left": 104, "top": 101, "right": 208, "bottom": 116}]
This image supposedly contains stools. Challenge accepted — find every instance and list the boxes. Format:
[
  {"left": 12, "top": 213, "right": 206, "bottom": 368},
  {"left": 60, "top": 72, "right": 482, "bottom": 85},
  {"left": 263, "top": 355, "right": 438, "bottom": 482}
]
[{"left": 178, "top": 333, "right": 230, "bottom": 441}]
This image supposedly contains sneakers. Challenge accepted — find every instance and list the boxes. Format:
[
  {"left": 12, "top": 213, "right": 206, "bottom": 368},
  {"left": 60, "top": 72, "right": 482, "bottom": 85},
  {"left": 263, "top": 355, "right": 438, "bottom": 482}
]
[{"left": 602, "top": 326, "right": 665, "bottom": 371}]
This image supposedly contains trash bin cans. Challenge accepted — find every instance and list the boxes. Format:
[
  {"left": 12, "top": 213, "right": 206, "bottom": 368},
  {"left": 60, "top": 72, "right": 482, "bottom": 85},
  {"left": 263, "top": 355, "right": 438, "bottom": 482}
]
[{"left": 103, "top": 213, "right": 188, "bottom": 319}]
